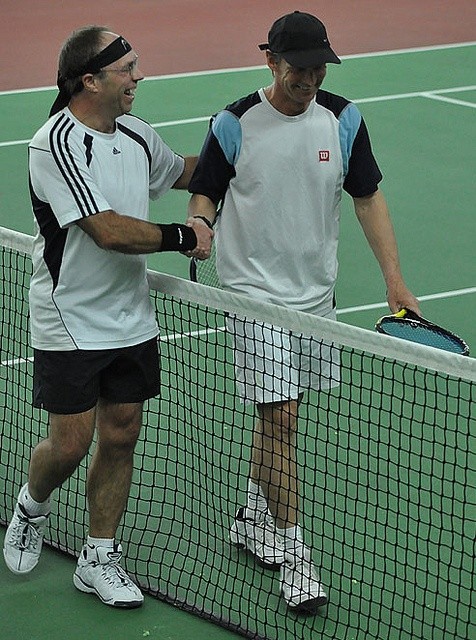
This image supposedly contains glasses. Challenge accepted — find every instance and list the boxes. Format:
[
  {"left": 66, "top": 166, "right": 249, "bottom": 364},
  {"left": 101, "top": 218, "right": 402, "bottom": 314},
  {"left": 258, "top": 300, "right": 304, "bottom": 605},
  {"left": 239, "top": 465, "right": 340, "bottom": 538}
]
[{"left": 100, "top": 57, "right": 138, "bottom": 75}]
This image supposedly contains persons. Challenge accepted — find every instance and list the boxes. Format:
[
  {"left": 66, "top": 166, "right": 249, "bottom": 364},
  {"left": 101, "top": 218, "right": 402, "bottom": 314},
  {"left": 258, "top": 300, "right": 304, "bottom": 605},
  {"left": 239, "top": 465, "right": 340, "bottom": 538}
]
[
  {"left": 2, "top": 24, "right": 215, "bottom": 609},
  {"left": 180, "top": 13, "right": 424, "bottom": 611}
]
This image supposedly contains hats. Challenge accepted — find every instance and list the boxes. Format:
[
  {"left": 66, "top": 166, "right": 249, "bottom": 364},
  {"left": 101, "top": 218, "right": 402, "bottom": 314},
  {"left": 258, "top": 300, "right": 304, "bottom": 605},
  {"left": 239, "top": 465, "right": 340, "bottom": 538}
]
[{"left": 258, "top": 11, "right": 341, "bottom": 66}]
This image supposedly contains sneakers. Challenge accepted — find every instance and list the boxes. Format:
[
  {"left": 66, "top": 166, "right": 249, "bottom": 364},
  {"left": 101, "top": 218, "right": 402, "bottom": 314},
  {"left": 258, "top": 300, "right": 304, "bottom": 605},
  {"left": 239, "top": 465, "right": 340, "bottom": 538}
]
[
  {"left": 229, "top": 506, "right": 285, "bottom": 569},
  {"left": 73, "top": 543, "right": 144, "bottom": 609},
  {"left": 3, "top": 482, "right": 51, "bottom": 575},
  {"left": 278, "top": 547, "right": 327, "bottom": 610}
]
